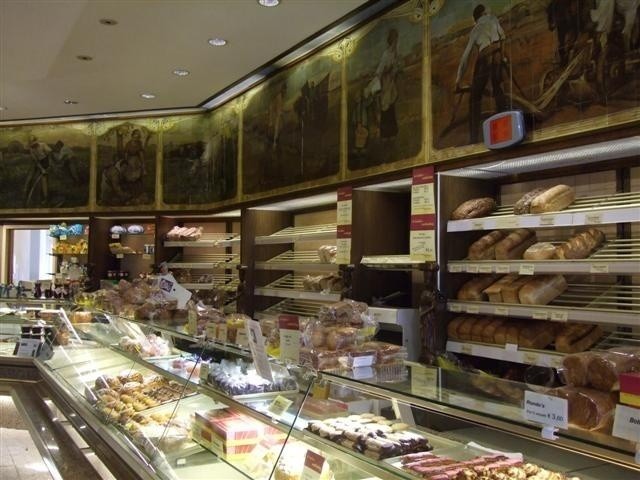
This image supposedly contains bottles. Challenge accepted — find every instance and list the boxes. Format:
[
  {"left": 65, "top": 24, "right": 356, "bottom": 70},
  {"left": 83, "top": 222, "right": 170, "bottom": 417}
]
[{"left": 0, "top": 278, "right": 81, "bottom": 298}]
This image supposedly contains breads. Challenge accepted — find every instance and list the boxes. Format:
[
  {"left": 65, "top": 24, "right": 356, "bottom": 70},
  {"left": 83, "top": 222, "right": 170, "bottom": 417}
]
[
  {"left": 514, "top": 184, "right": 575, "bottom": 216},
  {"left": 450, "top": 195, "right": 496, "bottom": 220},
  {"left": 84, "top": 369, "right": 193, "bottom": 426},
  {"left": 303, "top": 244, "right": 344, "bottom": 291},
  {"left": 468, "top": 227, "right": 605, "bottom": 260},
  {"left": 457, "top": 273, "right": 567, "bottom": 305},
  {"left": 447, "top": 314, "right": 640, "bottom": 432},
  {"left": 459, "top": 462, "right": 581, "bottom": 480},
  {"left": 307, "top": 412, "right": 433, "bottom": 460},
  {"left": 299, "top": 299, "right": 401, "bottom": 372}
]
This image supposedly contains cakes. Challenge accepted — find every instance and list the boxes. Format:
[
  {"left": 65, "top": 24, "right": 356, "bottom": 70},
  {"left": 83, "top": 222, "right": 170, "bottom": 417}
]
[{"left": 400, "top": 451, "right": 523, "bottom": 480}]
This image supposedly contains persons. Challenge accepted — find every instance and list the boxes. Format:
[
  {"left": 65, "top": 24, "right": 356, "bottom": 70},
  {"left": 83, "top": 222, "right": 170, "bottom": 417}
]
[
  {"left": 45, "top": 140, "right": 81, "bottom": 190},
  {"left": 262, "top": 83, "right": 290, "bottom": 157},
  {"left": 364, "top": 27, "right": 404, "bottom": 143},
  {"left": 101, "top": 129, "right": 148, "bottom": 204},
  {"left": 453, "top": 3, "right": 510, "bottom": 143},
  {"left": 23, "top": 139, "right": 55, "bottom": 202}
]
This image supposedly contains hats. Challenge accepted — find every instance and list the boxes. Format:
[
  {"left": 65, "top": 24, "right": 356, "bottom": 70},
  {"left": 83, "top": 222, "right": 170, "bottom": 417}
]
[
  {"left": 24, "top": 135, "right": 41, "bottom": 150},
  {"left": 48, "top": 141, "right": 64, "bottom": 152}
]
